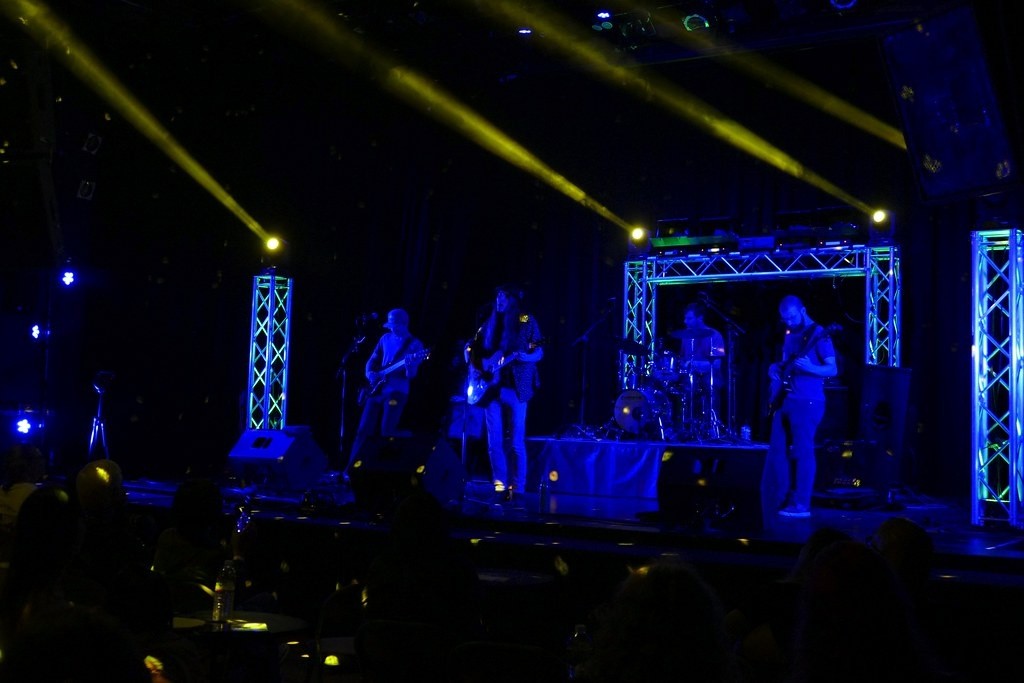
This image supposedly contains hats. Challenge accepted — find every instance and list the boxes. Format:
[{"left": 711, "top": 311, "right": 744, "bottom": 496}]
[{"left": 383, "top": 307, "right": 408, "bottom": 329}]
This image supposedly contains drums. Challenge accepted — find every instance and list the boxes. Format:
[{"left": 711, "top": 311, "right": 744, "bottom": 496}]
[
  {"left": 614, "top": 386, "right": 672, "bottom": 434},
  {"left": 678, "top": 360, "right": 711, "bottom": 374},
  {"left": 651, "top": 355, "right": 683, "bottom": 385}
]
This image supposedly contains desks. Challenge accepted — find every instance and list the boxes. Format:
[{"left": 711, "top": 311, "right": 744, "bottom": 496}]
[{"left": 187, "top": 609, "right": 309, "bottom": 682}]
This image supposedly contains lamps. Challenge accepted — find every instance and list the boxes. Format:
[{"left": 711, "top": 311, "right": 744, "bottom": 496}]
[
  {"left": 629, "top": 228, "right": 653, "bottom": 259},
  {"left": 349, "top": 459, "right": 378, "bottom": 501}
]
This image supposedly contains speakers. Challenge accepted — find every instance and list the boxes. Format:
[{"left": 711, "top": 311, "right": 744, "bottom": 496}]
[{"left": 228, "top": 429, "right": 326, "bottom": 491}]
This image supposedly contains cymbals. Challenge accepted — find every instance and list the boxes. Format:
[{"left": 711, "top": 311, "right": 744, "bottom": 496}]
[
  {"left": 670, "top": 327, "right": 716, "bottom": 340},
  {"left": 605, "top": 336, "right": 649, "bottom": 357}
]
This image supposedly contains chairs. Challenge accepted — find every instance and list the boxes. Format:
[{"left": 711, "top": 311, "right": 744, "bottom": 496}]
[{"left": 307, "top": 582, "right": 571, "bottom": 683}]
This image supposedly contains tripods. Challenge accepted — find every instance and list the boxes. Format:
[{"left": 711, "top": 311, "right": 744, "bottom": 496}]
[
  {"left": 670, "top": 337, "right": 741, "bottom": 446},
  {"left": 85, "top": 385, "right": 110, "bottom": 463}
]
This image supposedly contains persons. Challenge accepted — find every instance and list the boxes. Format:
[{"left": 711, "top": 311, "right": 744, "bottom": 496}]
[
  {"left": 679, "top": 301, "right": 723, "bottom": 430},
  {"left": 343, "top": 307, "right": 426, "bottom": 480},
  {"left": 0, "top": 445, "right": 959, "bottom": 683},
  {"left": 464, "top": 282, "right": 541, "bottom": 508},
  {"left": 768, "top": 295, "right": 839, "bottom": 511}
]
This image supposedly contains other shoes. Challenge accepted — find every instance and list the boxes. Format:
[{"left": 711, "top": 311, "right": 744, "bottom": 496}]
[
  {"left": 777, "top": 506, "right": 812, "bottom": 519},
  {"left": 512, "top": 492, "right": 523, "bottom": 501},
  {"left": 494, "top": 492, "right": 505, "bottom": 503}
]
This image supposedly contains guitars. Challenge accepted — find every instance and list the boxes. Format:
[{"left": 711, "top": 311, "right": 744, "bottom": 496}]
[
  {"left": 356, "top": 347, "right": 435, "bottom": 408},
  {"left": 768, "top": 321, "right": 845, "bottom": 407},
  {"left": 467, "top": 332, "right": 554, "bottom": 409}
]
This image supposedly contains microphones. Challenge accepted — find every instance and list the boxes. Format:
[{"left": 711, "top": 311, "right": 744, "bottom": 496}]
[{"left": 360, "top": 313, "right": 379, "bottom": 320}]
